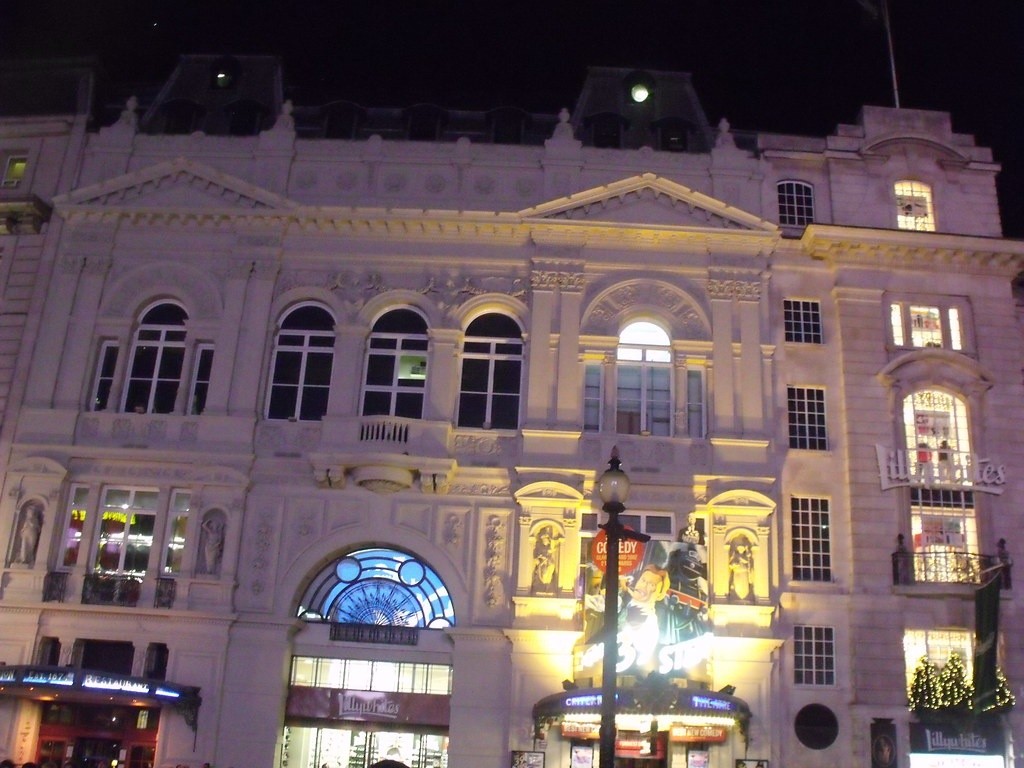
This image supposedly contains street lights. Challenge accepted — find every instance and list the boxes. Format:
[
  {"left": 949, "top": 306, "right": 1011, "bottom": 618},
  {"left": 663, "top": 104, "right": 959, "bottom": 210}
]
[{"left": 597, "top": 445, "right": 651, "bottom": 768}]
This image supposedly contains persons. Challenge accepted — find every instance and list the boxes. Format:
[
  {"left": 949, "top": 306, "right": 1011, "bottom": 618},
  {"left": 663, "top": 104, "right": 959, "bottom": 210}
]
[
  {"left": 939, "top": 441, "right": 952, "bottom": 460},
  {"left": 0, "top": 758, "right": 210, "bottom": 768},
  {"left": 202, "top": 519, "right": 223, "bottom": 574},
  {"left": 918, "top": 440, "right": 933, "bottom": 462},
  {"left": 727, "top": 543, "right": 755, "bottom": 603},
  {"left": 532, "top": 534, "right": 557, "bottom": 595},
  {"left": 21, "top": 507, "right": 39, "bottom": 564}
]
